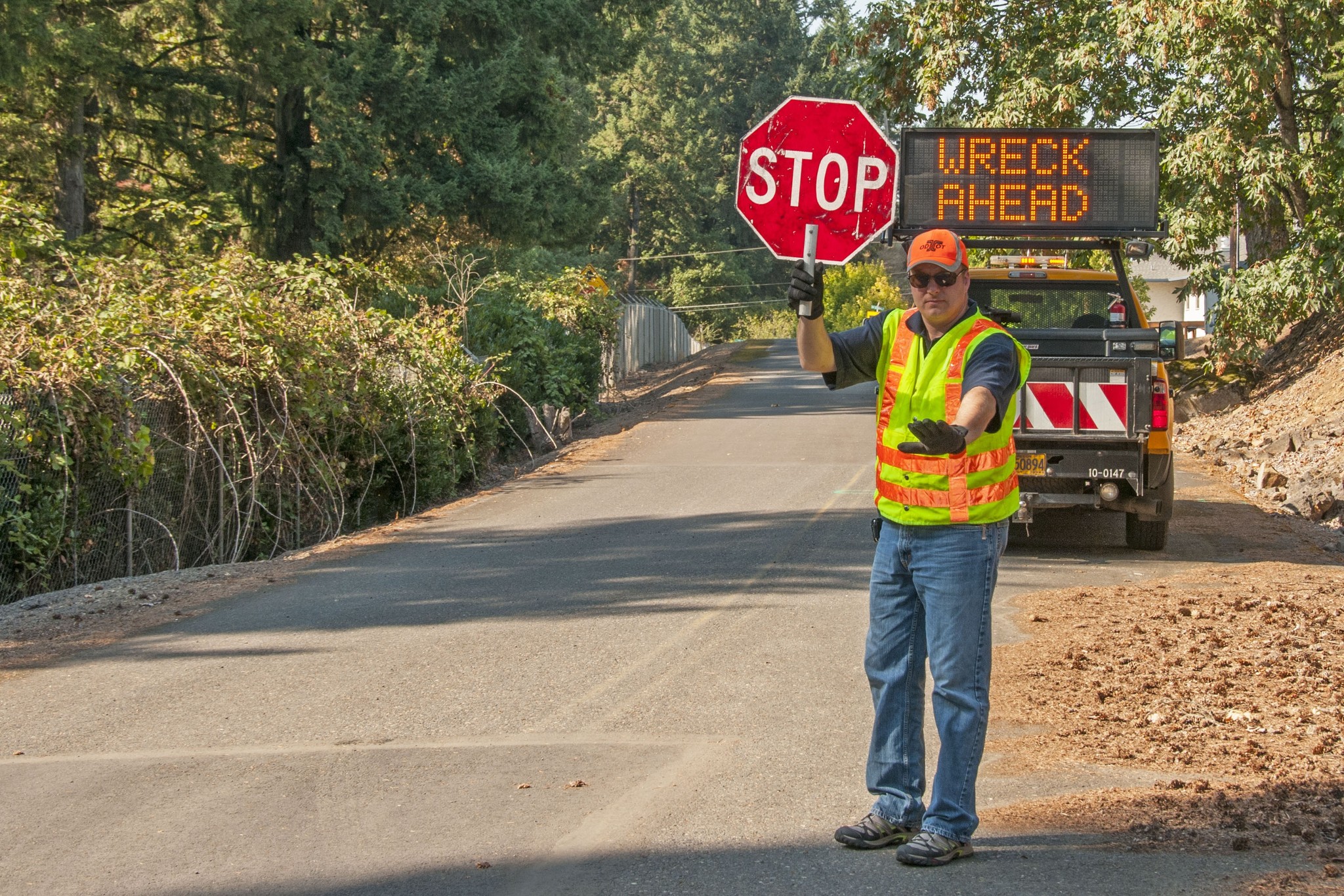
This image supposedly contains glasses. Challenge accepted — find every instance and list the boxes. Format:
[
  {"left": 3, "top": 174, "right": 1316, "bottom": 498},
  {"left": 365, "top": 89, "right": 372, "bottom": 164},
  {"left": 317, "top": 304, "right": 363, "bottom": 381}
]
[{"left": 906, "top": 267, "right": 970, "bottom": 289}]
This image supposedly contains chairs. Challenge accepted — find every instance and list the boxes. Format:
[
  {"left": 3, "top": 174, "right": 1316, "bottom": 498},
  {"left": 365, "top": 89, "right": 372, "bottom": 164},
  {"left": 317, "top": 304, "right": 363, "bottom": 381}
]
[{"left": 1072, "top": 314, "right": 1108, "bottom": 329}]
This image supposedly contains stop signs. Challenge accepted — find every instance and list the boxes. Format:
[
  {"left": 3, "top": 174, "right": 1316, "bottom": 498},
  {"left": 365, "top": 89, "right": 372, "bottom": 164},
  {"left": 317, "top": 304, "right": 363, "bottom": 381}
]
[{"left": 733, "top": 96, "right": 898, "bottom": 267}]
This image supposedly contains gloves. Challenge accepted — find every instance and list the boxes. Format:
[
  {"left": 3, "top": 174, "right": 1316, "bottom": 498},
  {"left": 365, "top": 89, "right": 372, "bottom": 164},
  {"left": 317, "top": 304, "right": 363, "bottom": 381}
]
[
  {"left": 897, "top": 419, "right": 968, "bottom": 455},
  {"left": 788, "top": 260, "right": 825, "bottom": 319}
]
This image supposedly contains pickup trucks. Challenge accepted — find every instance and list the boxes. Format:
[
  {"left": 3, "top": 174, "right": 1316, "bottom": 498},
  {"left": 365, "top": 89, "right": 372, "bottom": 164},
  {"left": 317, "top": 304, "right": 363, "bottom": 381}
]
[{"left": 935, "top": 239, "right": 1177, "bottom": 553}]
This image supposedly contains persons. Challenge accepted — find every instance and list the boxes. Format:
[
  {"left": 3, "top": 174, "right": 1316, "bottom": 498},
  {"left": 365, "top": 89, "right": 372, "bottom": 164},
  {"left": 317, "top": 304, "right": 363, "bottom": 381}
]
[
  {"left": 1161, "top": 349, "right": 1166, "bottom": 357},
  {"left": 787, "top": 229, "right": 1031, "bottom": 867}
]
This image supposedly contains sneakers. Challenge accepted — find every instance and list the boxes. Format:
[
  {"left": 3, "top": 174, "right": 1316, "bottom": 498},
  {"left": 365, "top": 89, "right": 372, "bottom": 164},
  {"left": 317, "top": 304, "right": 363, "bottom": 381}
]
[
  {"left": 833, "top": 813, "right": 923, "bottom": 849},
  {"left": 895, "top": 830, "right": 974, "bottom": 867}
]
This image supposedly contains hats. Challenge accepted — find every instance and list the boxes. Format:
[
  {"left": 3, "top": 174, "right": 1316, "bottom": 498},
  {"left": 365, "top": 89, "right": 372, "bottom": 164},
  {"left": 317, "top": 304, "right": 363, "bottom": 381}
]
[{"left": 906, "top": 230, "right": 967, "bottom": 274}]
